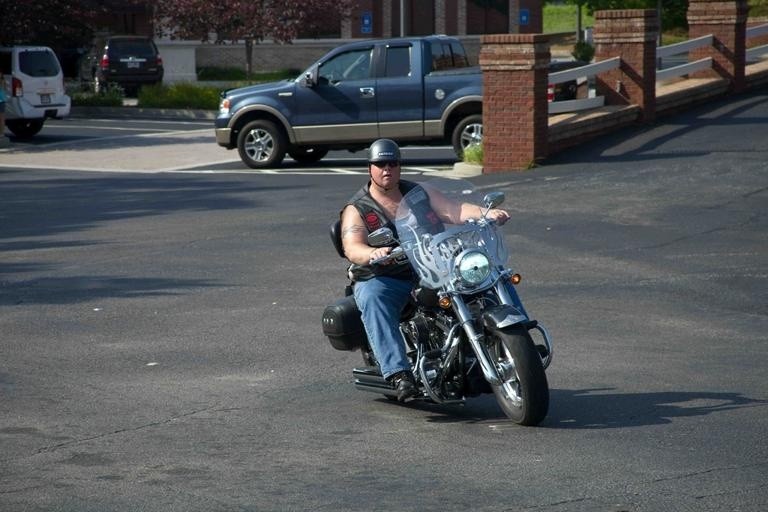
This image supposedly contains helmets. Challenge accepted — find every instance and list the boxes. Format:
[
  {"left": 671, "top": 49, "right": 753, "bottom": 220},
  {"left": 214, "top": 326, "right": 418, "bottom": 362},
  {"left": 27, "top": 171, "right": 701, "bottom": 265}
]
[{"left": 367, "top": 139, "right": 402, "bottom": 163}]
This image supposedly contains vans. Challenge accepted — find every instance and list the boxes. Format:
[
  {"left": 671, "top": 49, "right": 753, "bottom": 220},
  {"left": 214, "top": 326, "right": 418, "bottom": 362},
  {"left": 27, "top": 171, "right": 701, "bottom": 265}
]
[
  {"left": 77, "top": 33, "right": 168, "bottom": 97},
  {"left": 0, "top": 44, "right": 74, "bottom": 142}
]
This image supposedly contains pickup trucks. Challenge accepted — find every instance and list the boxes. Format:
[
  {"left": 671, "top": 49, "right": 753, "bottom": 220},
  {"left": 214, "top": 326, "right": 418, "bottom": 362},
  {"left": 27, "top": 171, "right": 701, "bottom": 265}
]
[{"left": 214, "top": 32, "right": 586, "bottom": 170}]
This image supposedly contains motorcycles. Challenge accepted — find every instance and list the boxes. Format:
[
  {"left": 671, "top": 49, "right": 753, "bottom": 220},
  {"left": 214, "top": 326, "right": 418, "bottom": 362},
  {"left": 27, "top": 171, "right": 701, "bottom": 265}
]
[{"left": 318, "top": 173, "right": 556, "bottom": 430}]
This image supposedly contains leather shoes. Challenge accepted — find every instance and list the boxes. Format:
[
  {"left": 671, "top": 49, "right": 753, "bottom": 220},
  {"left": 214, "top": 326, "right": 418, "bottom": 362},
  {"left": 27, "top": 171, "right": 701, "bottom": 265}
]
[{"left": 393, "top": 372, "right": 420, "bottom": 399}]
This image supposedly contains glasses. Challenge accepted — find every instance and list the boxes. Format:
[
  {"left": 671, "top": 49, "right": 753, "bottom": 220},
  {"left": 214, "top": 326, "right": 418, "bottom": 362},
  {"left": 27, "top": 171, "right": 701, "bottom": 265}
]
[{"left": 371, "top": 162, "right": 399, "bottom": 168}]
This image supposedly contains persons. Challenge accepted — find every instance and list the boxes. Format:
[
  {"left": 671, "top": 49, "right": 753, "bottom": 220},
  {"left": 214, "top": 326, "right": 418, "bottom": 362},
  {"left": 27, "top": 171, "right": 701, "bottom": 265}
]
[{"left": 341, "top": 137, "right": 511, "bottom": 401}]
